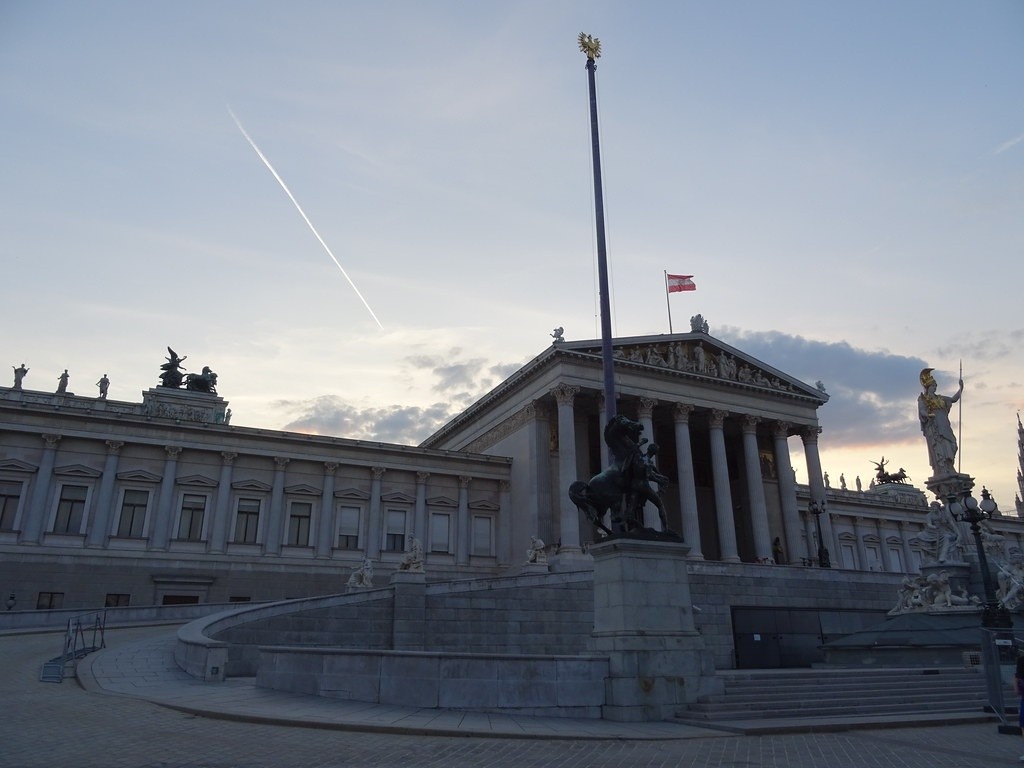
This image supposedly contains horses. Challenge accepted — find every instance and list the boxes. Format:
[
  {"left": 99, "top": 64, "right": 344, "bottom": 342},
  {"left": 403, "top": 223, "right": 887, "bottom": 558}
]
[
  {"left": 890, "top": 467, "right": 912, "bottom": 484},
  {"left": 568, "top": 415, "right": 669, "bottom": 543},
  {"left": 874, "top": 464, "right": 889, "bottom": 484},
  {"left": 183, "top": 366, "right": 218, "bottom": 394}
]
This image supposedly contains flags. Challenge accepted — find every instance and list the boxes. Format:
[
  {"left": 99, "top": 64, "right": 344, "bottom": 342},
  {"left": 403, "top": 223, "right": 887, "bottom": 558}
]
[{"left": 667, "top": 274, "right": 696, "bottom": 293}]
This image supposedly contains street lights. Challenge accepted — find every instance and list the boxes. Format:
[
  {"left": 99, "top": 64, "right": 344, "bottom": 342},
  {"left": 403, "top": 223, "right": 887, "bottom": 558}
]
[
  {"left": 808, "top": 499, "right": 831, "bottom": 569},
  {"left": 945, "top": 482, "right": 1020, "bottom": 668}
]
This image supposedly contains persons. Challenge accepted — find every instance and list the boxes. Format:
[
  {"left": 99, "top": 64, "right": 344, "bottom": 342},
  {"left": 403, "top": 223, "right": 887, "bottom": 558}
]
[
  {"left": 56, "top": 370, "right": 69, "bottom": 393},
  {"left": 979, "top": 519, "right": 1009, "bottom": 557},
  {"left": 824, "top": 471, "right": 830, "bottom": 488},
  {"left": 12, "top": 364, "right": 30, "bottom": 389},
  {"left": 1014, "top": 656, "right": 1024, "bottom": 761},
  {"left": 773, "top": 537, "right": 785, "bottom": 564},
  {"left": 521, "top": 536, "right": 545, "bottom": 566},
  {"left": 918, "top": 377, "right": 964, "bottom": 474},
  {"left": 619, "top": 438, "right": 679, "bottom": 535},
  {"left": 917, "top": 501, "right": 962, "bottom": 563},
  {"left": 690, "top": 314, "right": 709, "bottom": 333},
  {"left": 345, "top": 553, "right": 374, "bottom": 586},
  {"left": 897, "top": 573, "right": 952, "bottom": 607},
  {"left": 998, "top": 554, "right": 1024, "bottom": 604},
  {"left": 96, "top": 374, "right": 110, "bottom": 399},
  {"left": 615, "top": 342, "right": 794, "bottom": 391},
  {"left": 869, "top": 478, "right": 875, "bottom": 489},
  {"left": 398, "top": 533, "right": 423, "bottom": 570},
  {"left": 840, "top": 473, "right": 846, "bottom": 489},
  {"left": 225, "top": 409, "right": 232, "bottom": 424},
  {"left": 856, "top": 476, "right": 862, "bottom": 491},
  {"left": 753, "top": 557, "right": 763, "bottom": 564}
]
